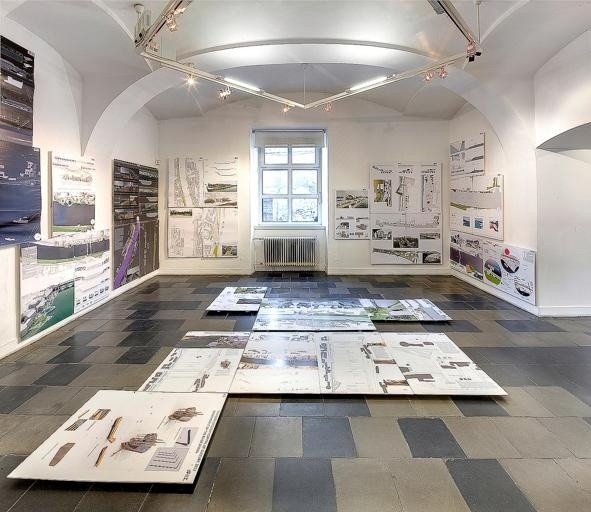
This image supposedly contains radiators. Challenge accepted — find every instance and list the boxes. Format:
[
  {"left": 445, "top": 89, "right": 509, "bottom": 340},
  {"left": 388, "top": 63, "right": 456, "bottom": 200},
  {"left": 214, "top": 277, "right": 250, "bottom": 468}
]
[{"left": 255, "top": 236, "right": 319, "bottom": 268}]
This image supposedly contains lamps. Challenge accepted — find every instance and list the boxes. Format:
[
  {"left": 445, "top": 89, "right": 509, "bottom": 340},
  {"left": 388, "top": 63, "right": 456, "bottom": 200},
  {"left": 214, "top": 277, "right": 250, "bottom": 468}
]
[
  {"left": 149, "top": 40, "right": 160, "bottom": 53},
  {"left": 165, "top": 11, "right": 179, "bottom": 32}
]
